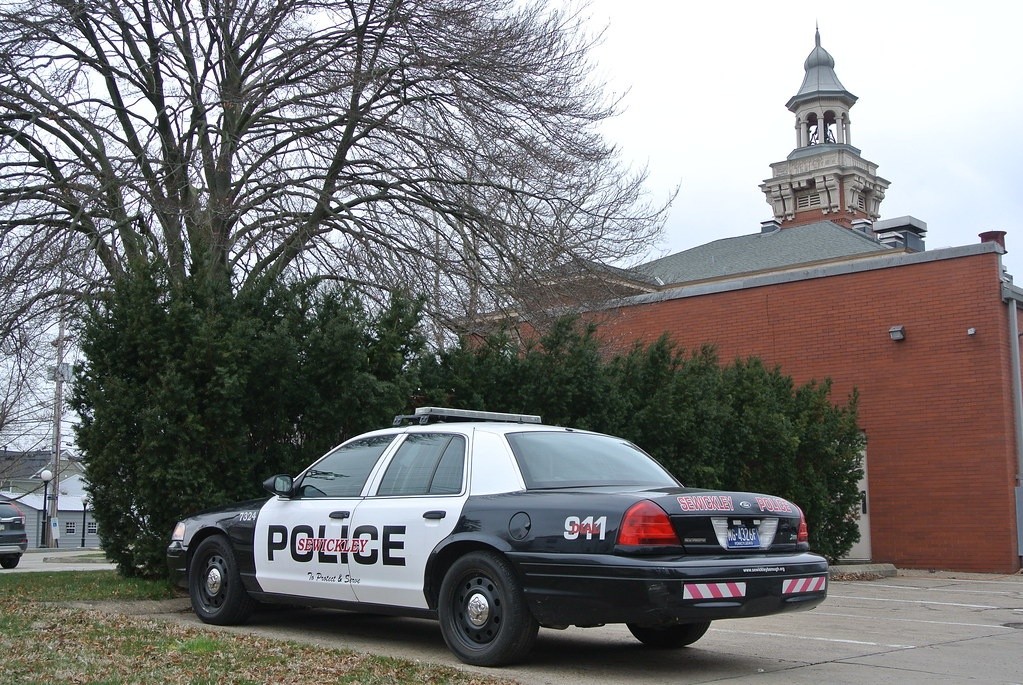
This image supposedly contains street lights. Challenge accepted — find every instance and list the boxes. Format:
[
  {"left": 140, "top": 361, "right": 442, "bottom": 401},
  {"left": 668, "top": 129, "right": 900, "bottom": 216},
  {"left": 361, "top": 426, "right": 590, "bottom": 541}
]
[
  {"left": 50, "top": 441, "right": 75, "bottom": 547},
  {"left": 79, "top": 494, "right": 90, "bottom": 548},
  {"left": 39, "top": 470, "right": 52, "bottom": 548}
]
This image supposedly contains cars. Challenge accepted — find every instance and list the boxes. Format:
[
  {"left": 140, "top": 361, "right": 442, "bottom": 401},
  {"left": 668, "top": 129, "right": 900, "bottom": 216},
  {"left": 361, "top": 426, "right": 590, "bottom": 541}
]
[
  {"left": 166, "top": 406, "right": 830, "bottom": 667},
  {"left": 0, "top": 501, "right": 29, "bottom": 569}
]
